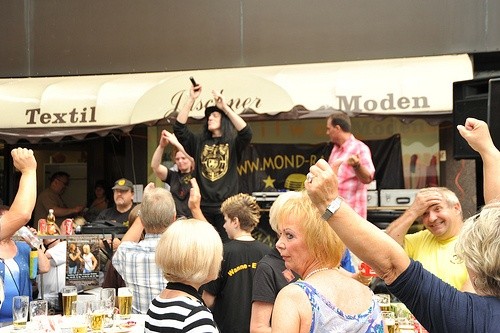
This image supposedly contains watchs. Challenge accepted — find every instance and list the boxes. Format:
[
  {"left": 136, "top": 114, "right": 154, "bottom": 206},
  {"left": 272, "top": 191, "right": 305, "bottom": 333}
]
[{"left": 320, "top": 196, "right": 343, "bottom": 220}]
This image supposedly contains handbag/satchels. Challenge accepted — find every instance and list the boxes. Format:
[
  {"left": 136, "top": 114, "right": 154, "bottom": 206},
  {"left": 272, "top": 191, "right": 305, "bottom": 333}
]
[{"left": 81, "top": 219, "right": 129, "bottom": 234}]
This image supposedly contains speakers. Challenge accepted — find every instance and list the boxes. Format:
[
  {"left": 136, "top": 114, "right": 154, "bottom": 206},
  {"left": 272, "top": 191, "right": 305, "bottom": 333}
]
[{"left": 453, "top": 75, "right": 500, "bottom": 160}]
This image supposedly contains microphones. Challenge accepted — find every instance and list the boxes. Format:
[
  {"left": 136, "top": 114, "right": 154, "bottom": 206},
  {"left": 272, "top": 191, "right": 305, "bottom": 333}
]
[{"left": 190, "top": 76, "right": 200, "bottom": 91}]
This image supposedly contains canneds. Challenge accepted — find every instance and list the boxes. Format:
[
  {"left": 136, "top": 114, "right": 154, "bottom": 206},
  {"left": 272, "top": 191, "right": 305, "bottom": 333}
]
[
  {"left": 38, "top": 219, "right": 47, "bottom": 235},
  {"left": 65, "top": 219, "right": 73, "bottom": 235}
]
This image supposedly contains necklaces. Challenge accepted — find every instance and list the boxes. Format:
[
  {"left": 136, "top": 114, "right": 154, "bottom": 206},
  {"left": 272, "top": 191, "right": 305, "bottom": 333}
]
[{"left": 304, "top": 266, "right": 339, "bottom": 280}]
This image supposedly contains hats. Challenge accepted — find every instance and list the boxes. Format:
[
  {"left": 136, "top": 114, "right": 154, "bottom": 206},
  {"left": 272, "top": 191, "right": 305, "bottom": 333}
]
[{"left": 112, "top": 179, "right": 134, "bottom": 190}]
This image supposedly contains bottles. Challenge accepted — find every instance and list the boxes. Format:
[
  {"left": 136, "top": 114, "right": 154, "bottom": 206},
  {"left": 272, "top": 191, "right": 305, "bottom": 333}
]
[{"left": 46, "top": 208, "right": 56, "bottom": 236}]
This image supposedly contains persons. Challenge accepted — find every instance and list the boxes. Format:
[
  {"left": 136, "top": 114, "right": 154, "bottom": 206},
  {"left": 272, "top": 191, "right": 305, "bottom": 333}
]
[
  {"left": 0, "top": 86, "right": 388, "bottom": 333},
  {"left": 387, "top": 187, "right": 479, "bottom": 295},
  {"left": 305, "top": 118, "right": 500, "bottom": 333}
]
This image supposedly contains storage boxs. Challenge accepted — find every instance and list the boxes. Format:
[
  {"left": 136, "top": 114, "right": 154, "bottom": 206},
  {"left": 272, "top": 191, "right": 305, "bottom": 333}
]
[{"left": 366, "top": 180, "right": 418, "bottom": 207}]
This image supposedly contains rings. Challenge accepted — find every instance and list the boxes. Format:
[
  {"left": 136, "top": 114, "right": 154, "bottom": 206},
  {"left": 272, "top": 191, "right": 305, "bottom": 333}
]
[{"left": 307, "top": 175, "right": 316, "bottom": 183}]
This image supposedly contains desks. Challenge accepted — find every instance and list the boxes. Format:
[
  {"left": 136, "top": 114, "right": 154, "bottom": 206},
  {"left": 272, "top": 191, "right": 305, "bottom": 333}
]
[{"left": 0, "top": 314, "right": 146, "bottom": 333}]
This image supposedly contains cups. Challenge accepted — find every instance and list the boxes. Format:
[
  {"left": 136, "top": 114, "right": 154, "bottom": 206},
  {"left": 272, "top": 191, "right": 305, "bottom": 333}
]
[
  {"left": 29, "top": 300, "right": 48, "bottom": 332},
  {"left": 72, "top": 325, "right": 87, "bottom": 333},
  {"left": 118, "top": 287, "right": 133, "bottom": 319},
  {"left": 12, "top": 296, "right": 29, "bottom": 330},
  {"left": 378, "top": 294, "right": 400, "bottom": 333},
  {"left": 102, "top": 288, "right": 117, "bottom": 319},
  {"left": 61, "top": 286, "right": 77, "bottom": 316},
  {"left": 88, "top": 311, "right": 106, "bottom": 333}
]
